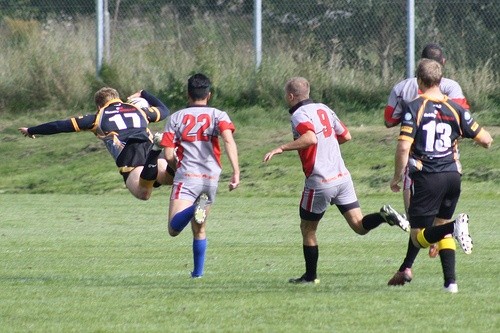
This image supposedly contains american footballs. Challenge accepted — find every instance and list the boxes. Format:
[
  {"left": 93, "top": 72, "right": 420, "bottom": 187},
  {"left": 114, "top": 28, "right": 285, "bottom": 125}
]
[{"left": 125, "top": 98, "right": 149, "bottom": 109}]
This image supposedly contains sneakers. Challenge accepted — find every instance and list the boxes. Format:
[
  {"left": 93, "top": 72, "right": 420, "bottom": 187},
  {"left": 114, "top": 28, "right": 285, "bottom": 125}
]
[
  {"left": 188, "top": 272, "right": 202, "bottom": 279},
  {"left": 174, "top": 148, "right": 179, "bottom": 161},
  {"left": 429, "top": 247, "right": 438, "bottom": 258},
  {"left": 380, "top": 205, "right": 411, "bottom": 233},
  {"left": 289, "top": 278, "right": 319, "bottom": 285},
  {"left": 194, "top": 193, "right": 208, "bottom": 224},
  {"left": 150, "top": 133, "right": 163, "bottom": 149},
  {"left": 454, "top": 213, "right": 474, "bottom": 255},
  {"left": 388, "top": 268, "right": 412, "bottom": 286},
  {"left": 442, "top": 283, "right": 458, "bottom": 293}
]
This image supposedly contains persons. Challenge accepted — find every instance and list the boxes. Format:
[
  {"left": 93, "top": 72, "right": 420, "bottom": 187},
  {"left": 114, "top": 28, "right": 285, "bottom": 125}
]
[
  {"left": 382, "top": 46, "right": 475, "bottom": 262},
  {"left": 17, "top": 88, "right": 174, "bottom": 201},
  {"left": 389, "top": 57, "right": 494, "bottom": 295},
  {"left": 262, "top": 77, "right": 411, "bottom": 286},
  {"left": 161, "top": 73, "right": 241, "bottom": 281}
]
